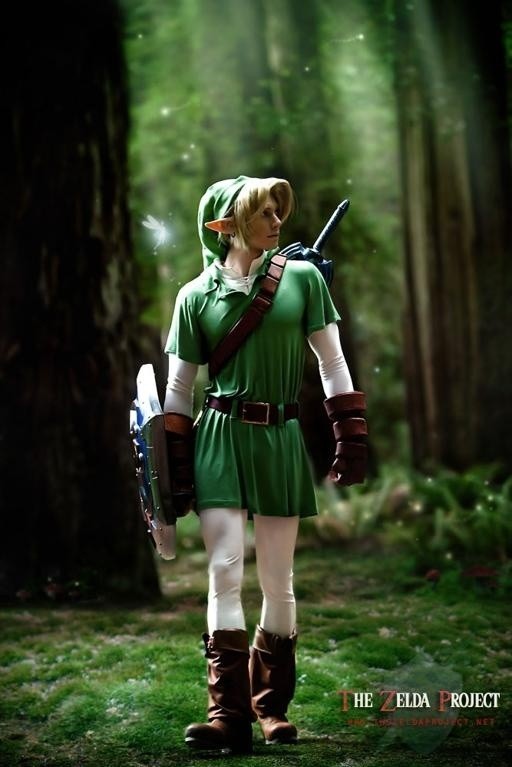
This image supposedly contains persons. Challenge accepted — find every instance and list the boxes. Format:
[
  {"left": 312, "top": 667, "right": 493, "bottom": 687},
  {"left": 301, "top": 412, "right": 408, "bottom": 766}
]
[{"left": 160, "top": 175, "right": 374, "bottom": 751}]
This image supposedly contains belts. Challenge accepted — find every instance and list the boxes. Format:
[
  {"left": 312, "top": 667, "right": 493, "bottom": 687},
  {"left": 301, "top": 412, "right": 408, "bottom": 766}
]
[{"left": 205, "top": 395, "right": 299, "bottom": 426}]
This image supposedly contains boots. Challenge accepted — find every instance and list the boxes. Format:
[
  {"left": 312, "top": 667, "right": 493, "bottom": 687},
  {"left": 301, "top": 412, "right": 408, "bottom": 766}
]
[
  {"left": 184, "top": 628, "right": 258, "bottom": 754},
  {"left": 248, "top": 622, "right": 299, "bottom": 745}
]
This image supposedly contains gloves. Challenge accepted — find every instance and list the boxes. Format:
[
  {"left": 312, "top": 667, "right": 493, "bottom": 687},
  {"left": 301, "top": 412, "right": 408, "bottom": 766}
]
[
  {"left": 324, "top": 391, "right": 372, "bottom": 488},
  {"left": 164, "top": 411, "right": 197, "bottom": 519}
]
